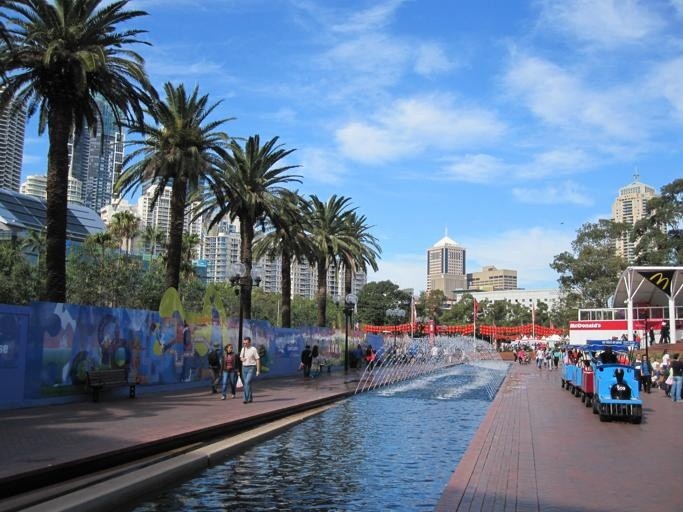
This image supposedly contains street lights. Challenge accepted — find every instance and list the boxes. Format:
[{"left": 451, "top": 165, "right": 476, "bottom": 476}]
[
  {"left": 332, "top": 293, "right": 358, "bottom": 375},
  {"left": 385, "top": 308, "right": 405, "bottom": 353},
  {"left": 224, "top": 261, "right": 262, "bottom": 381}
]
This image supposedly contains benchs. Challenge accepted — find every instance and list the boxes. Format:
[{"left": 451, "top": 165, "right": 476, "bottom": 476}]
[{"left": 87, "top": 367, "right": 140, "bottom": 403}]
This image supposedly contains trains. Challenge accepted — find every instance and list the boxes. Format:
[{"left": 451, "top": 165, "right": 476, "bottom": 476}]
[{"left": 559, "top": 341, "right": 643, "bottom": 423}]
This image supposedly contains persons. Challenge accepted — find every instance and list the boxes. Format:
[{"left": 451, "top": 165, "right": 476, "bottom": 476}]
[
  {"left": 206, "top": 341, "right": 223, "bottom": 391},
  {"left": 308, "top": 344, "right": 322, "bottom": 378},
  {"left": 300, "top": 344, "right": 312, "bottom": 378},
  {"left": 238, "top": 336, "right": 260, "bottom": 403},
  {"left": 510, "top": 320, "right": 682, "bottom": 403},
  {"left": 218, "top": 343, "right": 240, "bottom": 401},
  {"left": 354, "top": 343, "right": 452, "bottom": 371}
]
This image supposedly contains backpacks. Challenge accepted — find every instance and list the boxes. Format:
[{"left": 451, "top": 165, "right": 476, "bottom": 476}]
[{"left": 208, "top": 348, "right": 220, "bottom": 366}]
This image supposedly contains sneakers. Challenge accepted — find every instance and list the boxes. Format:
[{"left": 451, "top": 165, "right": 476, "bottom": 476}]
[
  {"left": 242, "top": 400, "right": 253, "bottom": 404},
  {"left": 221, "top": 395, "right": 235, "bottom": 400}
]
